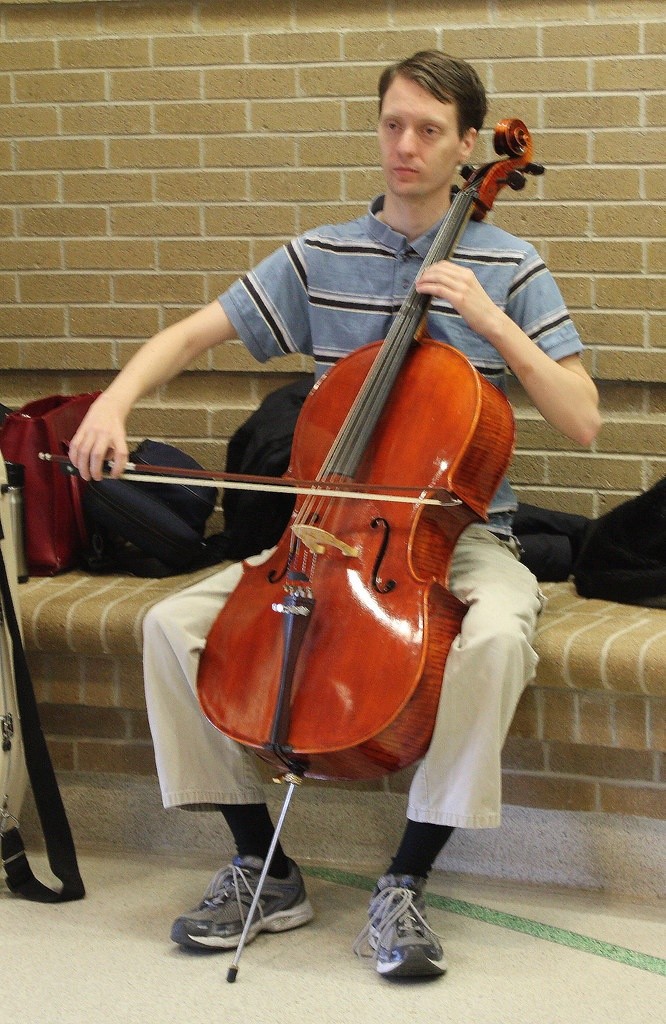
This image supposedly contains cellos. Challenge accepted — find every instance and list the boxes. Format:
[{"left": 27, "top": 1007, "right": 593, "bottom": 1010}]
[{"left": 192, "top": 116, "right": 547, "bottom": 982}]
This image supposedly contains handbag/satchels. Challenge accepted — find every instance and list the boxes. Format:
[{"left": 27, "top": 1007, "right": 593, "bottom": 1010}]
[
  {"left": 0, "top": 389, "right": 99, "bottom": 578},
  {"left": 82, "top": 438, "right": 218, "bottom": 580}
]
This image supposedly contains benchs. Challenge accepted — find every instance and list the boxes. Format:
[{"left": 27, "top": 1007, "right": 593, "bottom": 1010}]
[{"left": 15, "top": 556, "right": 666, "bottom": 900}]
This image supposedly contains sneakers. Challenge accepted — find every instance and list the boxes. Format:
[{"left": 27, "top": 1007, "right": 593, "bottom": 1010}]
[
  {"left": 353, "top": 874, "right": 447, "bottom": 978},
  {"left": 169, "top": 852, "right": 316, "bottom": 948}
]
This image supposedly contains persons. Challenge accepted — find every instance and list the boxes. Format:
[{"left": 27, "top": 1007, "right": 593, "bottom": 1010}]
[{"left": 69, "top": 50, "right": 599, "bottom": 975}]
